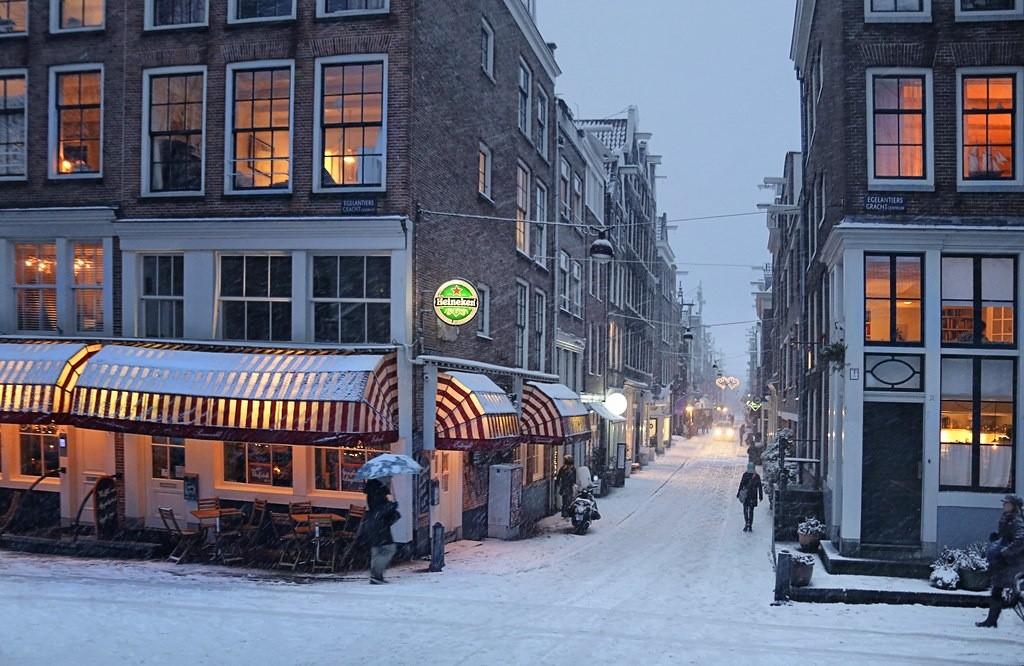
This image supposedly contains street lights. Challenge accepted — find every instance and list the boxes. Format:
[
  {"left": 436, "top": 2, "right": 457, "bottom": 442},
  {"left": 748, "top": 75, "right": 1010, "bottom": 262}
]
[{"left": 686, "top": 404, "right": 693, "bottom": 439}]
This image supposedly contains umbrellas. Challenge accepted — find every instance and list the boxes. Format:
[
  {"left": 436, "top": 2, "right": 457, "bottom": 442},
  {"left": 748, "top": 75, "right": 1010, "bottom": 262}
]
[{"left": 352, "top": 451, "right": 424, "bottom": 502}]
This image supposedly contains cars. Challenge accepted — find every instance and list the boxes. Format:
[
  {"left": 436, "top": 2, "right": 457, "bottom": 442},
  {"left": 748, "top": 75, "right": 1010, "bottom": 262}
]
[{"left": 713, "top": 421, "right": 734, "bottom": 441}]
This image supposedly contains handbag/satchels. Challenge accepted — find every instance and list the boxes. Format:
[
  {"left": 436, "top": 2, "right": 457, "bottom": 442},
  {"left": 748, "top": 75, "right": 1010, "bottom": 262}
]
[
  {"left": 559, "top": 485, "right": 565, "bottom": 496},
  {"left": 375, "top": 508, "right": 401, "bottom": 533},
  {"left": 739, "top": 490, "right": 747, "bottom": 503},
  {"left": 987, "top": 537, "right": 1003, "bottom": 556}
]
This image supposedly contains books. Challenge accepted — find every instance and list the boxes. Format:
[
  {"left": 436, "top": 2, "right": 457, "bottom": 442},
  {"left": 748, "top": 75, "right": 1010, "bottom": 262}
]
[
  {"left": 941, "top": 417, "right": 963, "bottom": 428},
  {"left": 942, "top": 307, "right": 972, "bottom": 342}
]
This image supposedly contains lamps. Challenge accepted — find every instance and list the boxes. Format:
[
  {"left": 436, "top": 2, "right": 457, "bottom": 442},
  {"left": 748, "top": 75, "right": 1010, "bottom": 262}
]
[{"left": 992, "top": 402, "right": 998, "bottom": 444}]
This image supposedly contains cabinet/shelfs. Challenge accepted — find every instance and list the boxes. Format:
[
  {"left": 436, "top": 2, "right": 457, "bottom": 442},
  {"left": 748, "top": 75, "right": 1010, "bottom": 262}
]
[
  {"left": 866, "top": 309, "right": 872, "bottom": 341},
  {"left": 942, "top": 305, "right": 973, "bottom": 343}
]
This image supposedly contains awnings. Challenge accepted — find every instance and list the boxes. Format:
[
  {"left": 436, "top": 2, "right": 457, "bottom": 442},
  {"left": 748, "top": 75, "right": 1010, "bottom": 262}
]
[
  {"left": 70, "top": 336, "right": 400, "bottom": 445},
  {"left": 518, "top": 380, "right": 593, "bottom": 447},
  {"left": 435, "top": 370, "right": 523, "bottom": 452},
  {"left": 585, "top": 401, "right": 628, "bottom": 423},
  {"left": 1, "top": 338, "right": 104, "bottom": 428}
]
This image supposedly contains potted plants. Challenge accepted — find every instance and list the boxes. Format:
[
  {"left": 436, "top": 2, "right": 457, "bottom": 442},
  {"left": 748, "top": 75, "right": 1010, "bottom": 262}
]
[
  {"left": 789, "top": 553, "right": 815, "bottom": 588},
  {"left": 818, "top": 343, "right": 851, "bottom": 377},
  {"left": 796, "top": 517, "right": 826, "bottom": 553},
  {"left": 958, "top": 545, "right": 994, "bottom": 591}
]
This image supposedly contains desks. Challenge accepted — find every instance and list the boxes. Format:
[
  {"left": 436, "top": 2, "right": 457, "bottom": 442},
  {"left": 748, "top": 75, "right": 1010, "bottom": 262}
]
[
  {"left": 189, "top": 508, "right": 241, "bottom": 555},
  {"left": 290, "top": 513, "right": 345, "bottom": 565}
]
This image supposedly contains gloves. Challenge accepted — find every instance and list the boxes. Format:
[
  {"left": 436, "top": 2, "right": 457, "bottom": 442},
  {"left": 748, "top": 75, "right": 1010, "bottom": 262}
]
[
  {"left": 737, "top": 493, "right": 739, "bottom": 498},
  {"left": 995, "top": 552, "right": 1004, "bottom": 560},
  {"left": 759, "top": 497, "right": 763, "bottom": 501},
  {"left": 990, "top": 532, "right": 998, "bottom": 541}
]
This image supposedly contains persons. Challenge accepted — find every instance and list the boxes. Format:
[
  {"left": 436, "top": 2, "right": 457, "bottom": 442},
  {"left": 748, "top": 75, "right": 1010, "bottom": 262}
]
[
  {"left": 364, "top": 475, "right": 399, "bottom": 585},
  {"left": 739, "top": 423, "right": 746, "bottom": 446},
  {"left": 557, "top": 455, "right": 577, "bottom": 522},
  {"left": 736, "top": 461, "right": 763, "bottom": 532},
  {"left": 692, "top": 407, "right": 735, "bottom": 435},
  {"left": 953, "top": 319, "right": 991, "bottom": 341},
  {"left": 975, "top": 493, "right": 1024, "bottom": 627}
]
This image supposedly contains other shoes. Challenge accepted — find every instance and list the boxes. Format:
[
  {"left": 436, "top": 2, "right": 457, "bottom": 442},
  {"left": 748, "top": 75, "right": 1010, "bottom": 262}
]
[
  {"left": 743, "top": 524, "right": 752, "bottom": 532},
  {"left": 975, "top": 617, "right": 997, "bottom": 628},
  {"left": 369, "top": 576, "right": 389, "bottom": 585}
]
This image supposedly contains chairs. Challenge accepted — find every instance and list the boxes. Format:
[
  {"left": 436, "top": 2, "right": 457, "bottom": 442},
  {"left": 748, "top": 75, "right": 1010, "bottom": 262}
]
[{"left": 158, "top": 497, "right": 367, "bottom": 574}]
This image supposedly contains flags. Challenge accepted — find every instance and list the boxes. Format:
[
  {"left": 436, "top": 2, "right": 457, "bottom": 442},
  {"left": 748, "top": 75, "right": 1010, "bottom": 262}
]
[
  {"left": 673, "top": 397, "right": 687, "bottom": 416},
  {"left": 655, "top": 384, "right": 672, "bottom": 407}
]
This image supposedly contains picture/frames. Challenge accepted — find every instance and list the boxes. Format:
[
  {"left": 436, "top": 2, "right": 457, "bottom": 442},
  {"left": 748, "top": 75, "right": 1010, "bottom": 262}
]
[
  {"left": 247, "top": 134, "right": 275, "bottom": 179},
  {"left": 248, "top": 463, "right": 271, "bottom": 485}
]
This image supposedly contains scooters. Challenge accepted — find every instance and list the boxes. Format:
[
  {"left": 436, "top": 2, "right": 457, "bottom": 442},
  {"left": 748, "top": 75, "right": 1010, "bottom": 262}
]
[{"left": 568, "top": 475, "right": 601, "bottom": 535}]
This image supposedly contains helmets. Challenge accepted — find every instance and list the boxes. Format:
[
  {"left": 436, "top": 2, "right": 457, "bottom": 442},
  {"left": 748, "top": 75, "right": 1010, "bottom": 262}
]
[{"left": 564, "top": 455, "right": 573, "bottom": 464}]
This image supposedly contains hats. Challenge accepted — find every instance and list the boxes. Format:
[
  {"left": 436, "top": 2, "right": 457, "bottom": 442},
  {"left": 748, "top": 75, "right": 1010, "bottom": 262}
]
[
  {"left": 748, "top": 464, "right": 755, "bottom": 473},
  {"left": 1000, "top": 494, "right": 1024, "bottom": 507}
]
